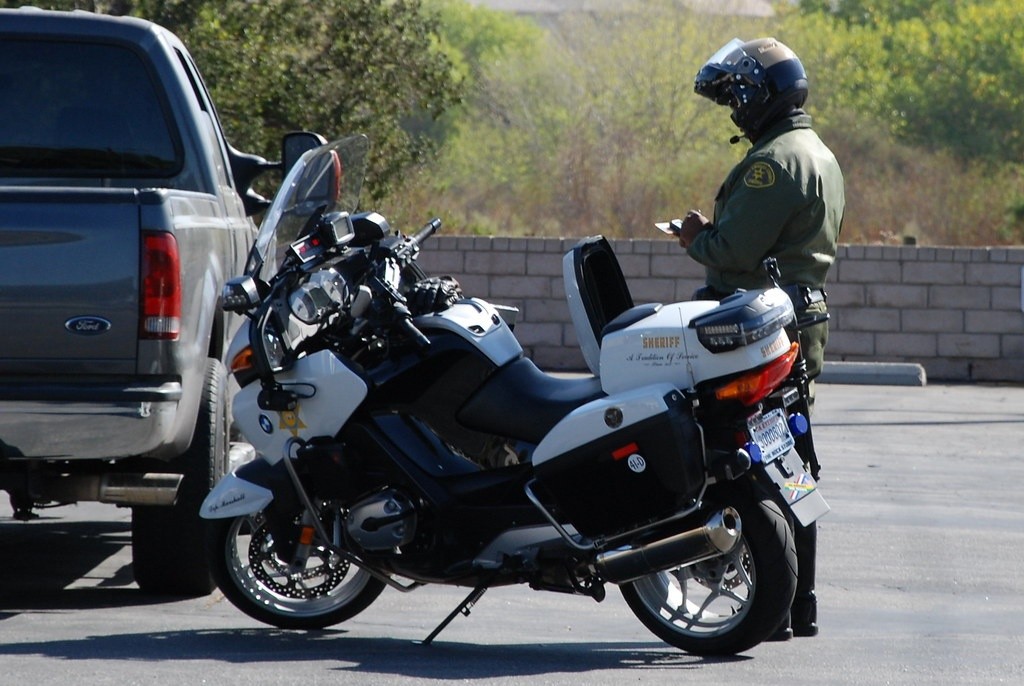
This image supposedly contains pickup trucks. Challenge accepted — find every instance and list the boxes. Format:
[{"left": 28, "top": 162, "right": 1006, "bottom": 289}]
[{"left": 0, "top": 6, "right": 331, "bottom": 604}]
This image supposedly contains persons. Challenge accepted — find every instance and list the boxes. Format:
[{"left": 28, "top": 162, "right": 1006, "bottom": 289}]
[{"left": 679, "top": 34, "right": 848, "bottom": 639}]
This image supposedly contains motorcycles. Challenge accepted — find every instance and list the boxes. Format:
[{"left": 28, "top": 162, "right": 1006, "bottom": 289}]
[{"left": 196, "top": 135, "right": 830, "bottom": 659}]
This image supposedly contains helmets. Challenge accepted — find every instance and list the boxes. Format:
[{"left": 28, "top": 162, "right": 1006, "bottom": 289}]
[{"left": 693, "top": 37, "right": 809, "bottom": 142}]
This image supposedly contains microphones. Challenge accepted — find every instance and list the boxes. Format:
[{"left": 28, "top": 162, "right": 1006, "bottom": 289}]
[{"left": 729, "top": 134, "right": 747, "bottom": 144}]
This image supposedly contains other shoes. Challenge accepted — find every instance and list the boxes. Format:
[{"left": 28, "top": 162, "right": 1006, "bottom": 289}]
[
  {"left": 791, "top": 591, "right": 819, "bottom": 637},
  {"left": 764, "top": 610, "right": 792, "bottom": 642}
]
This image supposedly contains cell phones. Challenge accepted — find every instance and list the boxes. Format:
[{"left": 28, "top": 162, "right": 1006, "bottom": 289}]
[{"left": 670, "top": 217, "right": 682, "bottom": 235}]
